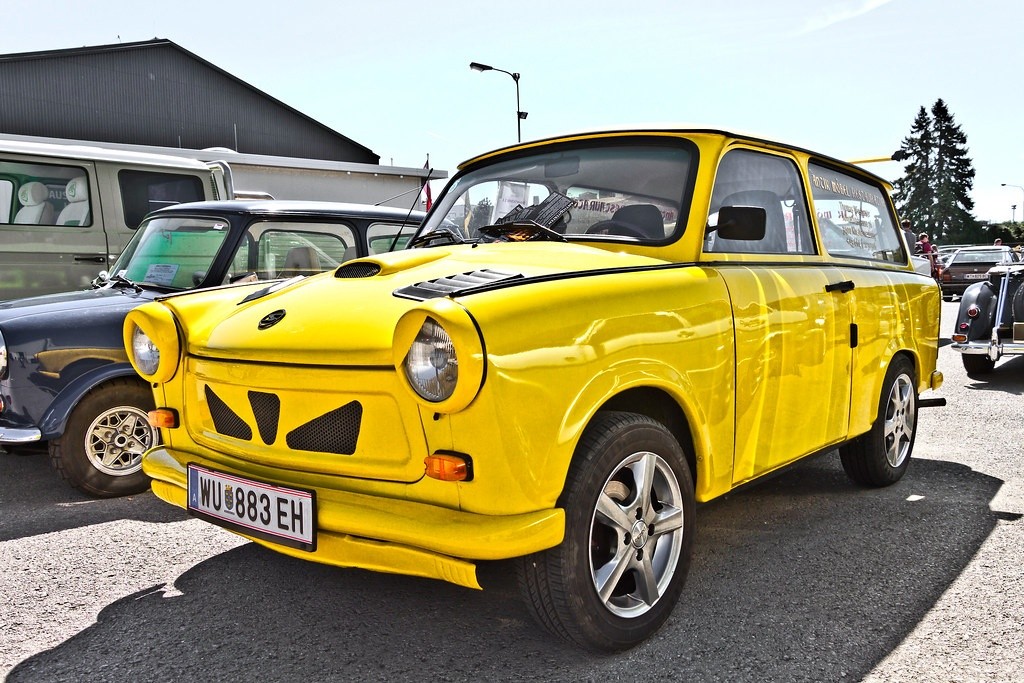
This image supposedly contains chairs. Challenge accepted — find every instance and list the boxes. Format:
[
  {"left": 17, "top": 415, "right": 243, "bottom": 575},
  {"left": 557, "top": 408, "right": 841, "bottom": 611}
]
[
  {"left": 609, "top": 204, "right": 664, "bottom": 239},
  {"left": 282, "top": 247, "right": 322, "bottom": 277},
  {"left": 12, "top": 181, "right": 54, "bottom": 224},
  {"left": 709, "top": 190, "right": 788, "bottom": 252},
  {"left": 55, "top": 175, "right": 90, "bottom": 226},
  {"left": 342, "top": 246, "right": 375, "bottom": 265}
]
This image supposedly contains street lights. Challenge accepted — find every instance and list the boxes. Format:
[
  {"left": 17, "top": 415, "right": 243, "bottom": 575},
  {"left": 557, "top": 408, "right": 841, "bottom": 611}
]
[
  {"left": 470, "top": 61, "right": 522, "bottom": 143},
  {"left": 1000, "top": 182, "right": 1024, "bottom": 224}
]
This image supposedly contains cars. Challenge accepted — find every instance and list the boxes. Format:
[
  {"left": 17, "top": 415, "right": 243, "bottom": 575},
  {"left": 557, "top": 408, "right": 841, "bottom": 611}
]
[
  {"left": 0, "top": 194, "right": 470, "bottom": 502},
  {"left": 933, "top": 243, "right": 1024, "bottom": 377}
]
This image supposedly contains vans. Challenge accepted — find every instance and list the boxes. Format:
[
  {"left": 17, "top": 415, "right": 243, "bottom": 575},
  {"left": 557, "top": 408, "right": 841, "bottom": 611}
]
[{"left": 0, "top": 135, "right": 277, "bottom": 304}]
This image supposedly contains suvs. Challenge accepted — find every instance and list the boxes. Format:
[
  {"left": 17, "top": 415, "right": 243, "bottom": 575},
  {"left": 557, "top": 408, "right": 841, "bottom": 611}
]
[{"left": 122, "top": 125, "right": 948, "bottom": 654}]
